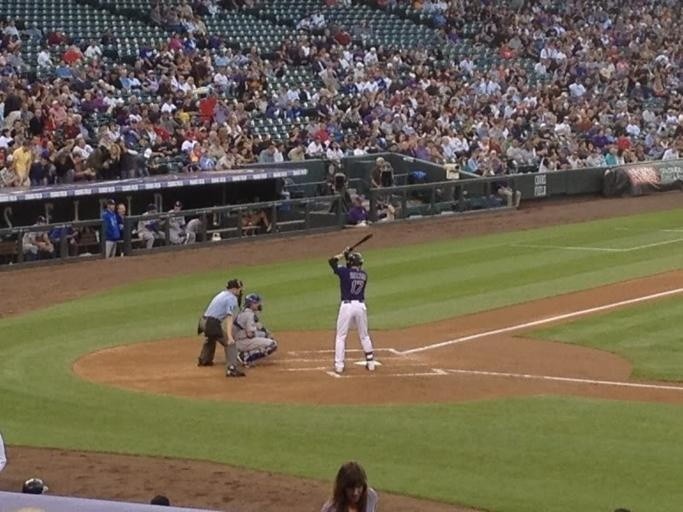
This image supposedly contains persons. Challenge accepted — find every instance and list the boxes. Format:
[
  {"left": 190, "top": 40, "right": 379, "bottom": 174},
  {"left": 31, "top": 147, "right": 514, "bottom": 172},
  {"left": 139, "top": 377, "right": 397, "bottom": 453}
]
[
  {"left": 494, "top": 163, "right": 521, "bottom": 209},
  {"left": 242, "top": 209, "right": 269, "bottom": 237},
  {"left": 51, "top": 225, "right": 79, "bottom": 257},
  {"left": 23, "top": 216, "right": 54, "bottom": 262},
  {"left": 194, "top": 277, "right": 246, "bottom": 377},
  {"left": 370, "top": 156, "right": 400, "bottom": 208},
  {"left": 326, "top": 245, "right": 378, "bottom": 376},
  {"left": 116, "top": 203, "right": 137, "bottom": 257},
  {"left": 228, "top": 294, "right": 278, "bottom": 366},
  {"left": 346, "top": 194, "right": 369, "bottom": 227},
  {"left": 0, "top": 2, "right": 683, "bottom": 262},
  {"left": 329, "top": 172, "right": 352, "bottom": 213},
  {"left": 320, "top": 161, "right": 337, "bottom": 195},
  {"left": 138, "top": 204, "right": 166, "bottom": 249},
  {"left": 166, "top": 201, "right": 187, "bottom": 245},
  {"left": 102, "top": 199, "right": 124, "bottom": 260},
  {"left": 319, "top": 461, "right": 378, "bottom": 512}
]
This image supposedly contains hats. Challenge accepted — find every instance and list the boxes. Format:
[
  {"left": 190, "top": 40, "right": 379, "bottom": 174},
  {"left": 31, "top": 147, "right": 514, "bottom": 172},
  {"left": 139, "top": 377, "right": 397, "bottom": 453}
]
[
  {"left": 36, "top": 215, "right": 47, "bottom": 223},
  {"left": 105, "top": 199, "right": 116, "bottom": 205},
  {"left": 228, "top": 278, "right": 244, "bottom": 289}
]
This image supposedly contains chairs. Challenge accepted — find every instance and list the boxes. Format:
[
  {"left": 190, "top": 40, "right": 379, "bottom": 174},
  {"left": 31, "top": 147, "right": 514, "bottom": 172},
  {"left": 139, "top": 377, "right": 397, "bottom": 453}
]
[{"left": 0, "top": 0, "right": 667, "bottom": 264}]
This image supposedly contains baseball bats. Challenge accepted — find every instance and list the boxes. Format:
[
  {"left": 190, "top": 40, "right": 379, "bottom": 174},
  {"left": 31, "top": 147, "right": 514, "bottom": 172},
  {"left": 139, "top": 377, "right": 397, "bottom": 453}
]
[{"left": 349, "top": 233, "right": 372, "bottom": 251}]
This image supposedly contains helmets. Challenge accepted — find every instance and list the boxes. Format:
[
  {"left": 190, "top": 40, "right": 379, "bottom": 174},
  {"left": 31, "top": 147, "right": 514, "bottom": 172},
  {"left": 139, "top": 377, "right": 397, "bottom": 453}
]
[
  {"left": 346, "top": 252, "right": 364, "bottom": 267},
  {"left": 245, "top": 294, "right": 262, "bottom": 312},
  {"left": 22, "top": 478, "right": 48, "bottom": 494}
]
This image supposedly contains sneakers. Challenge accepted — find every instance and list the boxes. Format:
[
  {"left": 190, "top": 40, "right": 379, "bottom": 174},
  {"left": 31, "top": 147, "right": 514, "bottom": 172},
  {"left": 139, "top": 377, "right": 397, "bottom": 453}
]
[
  {"left": 225, "top": 365, "right": 246, "bottom": 377},
  {"left": 334, "top": 367, "right": 345, "bottom": 374},
  {"left": 365, "top": 361, "right": 376, "bottom": 372},
  {"left": 197, "top": 357, "right": 215, "bottom": 366}
]
